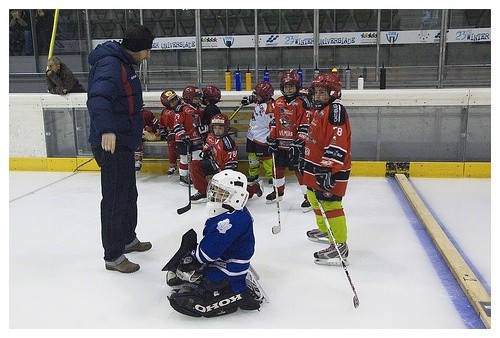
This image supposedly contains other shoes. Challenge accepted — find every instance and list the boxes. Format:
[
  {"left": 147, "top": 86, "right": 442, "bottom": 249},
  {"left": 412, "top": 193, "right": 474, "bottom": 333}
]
[
  {"left": 123, "top": 241, "right": 152, "bottom": 253},
  {"left": 106, "top": 258, "right": 140, "bottom": 273}
]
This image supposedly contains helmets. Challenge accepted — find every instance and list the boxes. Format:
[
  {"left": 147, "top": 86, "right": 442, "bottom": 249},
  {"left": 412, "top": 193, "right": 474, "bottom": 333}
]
[
  {"left": 253, "top": 81, "right": 274, "bottom": 99},
  {"left": 160, "top": 89, "right": 177, "bottom": 106},
  {"left": 211, "top": 114, "right": 231, "bottom": 135},
  {"left": 205, "top": 169, "right": 249, "bottom": 218},
  {"left": 310, "top": 73, "right": 341, "bottom": 110},
  {"left": 279, "top": 71, "right": 300, "bottom": 97},
  {"left": 203, "top": 86, "right": 222, "bottom": 100},
  {"left": 183, "top": 86, "right": 202, "bottom": 100}
]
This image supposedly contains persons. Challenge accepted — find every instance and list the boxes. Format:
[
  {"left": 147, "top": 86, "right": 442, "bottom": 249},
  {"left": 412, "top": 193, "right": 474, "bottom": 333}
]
[
  {"left": 135, "top": 84, "right": 223, "bottom": 188},
  {"left": 46, "top": 56, "right": 86, "bottom": 95},
  {"left": 242, "top": 81, "right": 277, "bottom": 186},
  {"left": 33, "top": 9, "right": 54, "bottom": 55},
  {"left": 189, "top": 113, "right": 262, "bottom": 200},
  {"left": 9, "top": 9, "right": 30, "bottom": 56},
  {"left": 302, "top": 73, "right": 352, "bottom": 266},
  {"left": 266, "top": 71, "right": 319, "bottom": 212},
  {"left": 162, "top": 169, "right": 263, "bottom": 317},
  {"left": 303, "top": 81, "right": 322, "bottom": 108},
  {"left": 86, "top": 23, "right": 154, "bottom": 273}
]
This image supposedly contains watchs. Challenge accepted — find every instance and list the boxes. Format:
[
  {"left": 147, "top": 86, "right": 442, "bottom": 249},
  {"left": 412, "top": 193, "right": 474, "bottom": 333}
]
[{"left": 46, "top": 69, "right": 51, "bottom": 74}]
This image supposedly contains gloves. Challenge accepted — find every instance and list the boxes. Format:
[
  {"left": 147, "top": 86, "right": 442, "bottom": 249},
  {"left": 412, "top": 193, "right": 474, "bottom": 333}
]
[
  {"left": 286, "top": 141, "right": 301, "bottom": 166},
  {"left": 315, "top": 166, "right": 336, "bottom": 192},
  {"left": 241, "top": 97, "right": 252, "bottom": 105},
  {"left": 266, "top": 136, "right": 279, "bottom": 154},
  {"left": 176, "top": 244, "right": 202, "bottom": 283}
]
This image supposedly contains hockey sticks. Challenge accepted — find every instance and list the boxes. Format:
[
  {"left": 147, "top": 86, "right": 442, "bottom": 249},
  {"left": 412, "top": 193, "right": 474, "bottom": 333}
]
[
  {"left": 229, "top": 104, "right": 243, "bottom": 121},
  {"left": 318, "top": 200, "right": 359, "bottom": 309},
  {"left": 272, "top": 152, "right": 281, "bottom": 235},
  {"left": 177, "top": 142, "right": 191, "bottom": 215},
  {"left": 209, "top": 156, "right": 221, "bottom": 173}
]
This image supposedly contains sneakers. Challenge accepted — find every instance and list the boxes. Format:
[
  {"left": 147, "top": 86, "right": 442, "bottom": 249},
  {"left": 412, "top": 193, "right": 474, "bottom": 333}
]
[
  {"left": 301, "top": 193, "right": 312, "bottom": 214},
  {"left": 266, "top": 186, "right": 284, "bottom": 204},
  {"left": 167, "top": 163, "right": 177, "bottom": 178},
  {"left": 134, "top": 160, "right": 143, "bottom": 175},
  {"left": 306, "top": 229, "right": 329, "bottom": 241},
  {"left": 314, "top": 242, "right": 350, "bottom": 266},
  {"left": 245, "top": 266, "right": 270, "bottom": 303},
  {"left": 190, "top": 190, "right": 207, "bottom": 203},
  {"left": 180, "top": 175, "right": 193, "bottom": 186}
]
